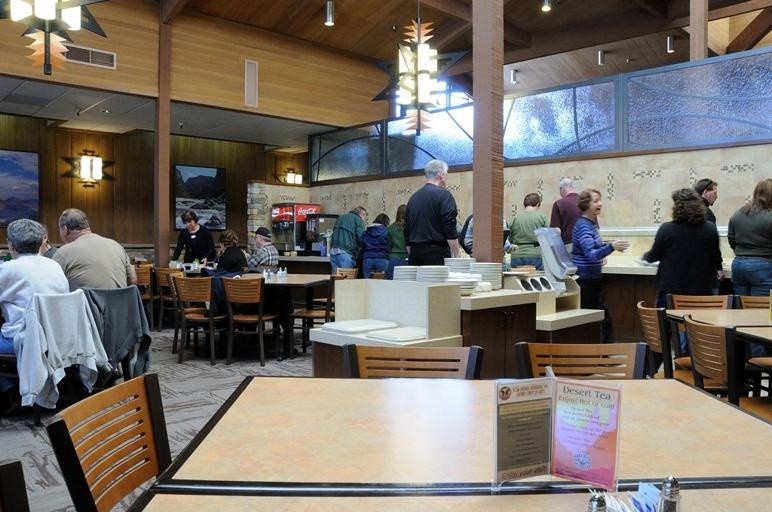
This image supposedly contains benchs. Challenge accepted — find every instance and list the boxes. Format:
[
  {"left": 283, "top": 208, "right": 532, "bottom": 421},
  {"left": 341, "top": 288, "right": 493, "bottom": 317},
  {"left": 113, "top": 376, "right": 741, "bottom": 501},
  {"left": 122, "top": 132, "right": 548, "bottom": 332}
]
[{"left": 307, "top": 277, "right": 463, "bottom": 377}]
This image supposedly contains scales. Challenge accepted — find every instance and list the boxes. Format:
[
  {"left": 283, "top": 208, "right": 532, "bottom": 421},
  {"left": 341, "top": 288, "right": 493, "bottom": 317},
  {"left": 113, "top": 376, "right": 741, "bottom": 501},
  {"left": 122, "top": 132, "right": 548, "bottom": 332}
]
[{"left": 504, "top": 228, "right": 579, "bottom": 296}]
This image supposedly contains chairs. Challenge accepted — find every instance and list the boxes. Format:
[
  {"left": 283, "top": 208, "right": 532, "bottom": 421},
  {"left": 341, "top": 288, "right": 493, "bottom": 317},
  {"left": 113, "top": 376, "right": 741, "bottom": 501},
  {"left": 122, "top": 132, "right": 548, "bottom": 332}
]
[
  {"left": 136, "top": 261, "right": 386, "bottom": 369},
  {"left": 635, "top": 292, "right": 771, "bottom": 422}
]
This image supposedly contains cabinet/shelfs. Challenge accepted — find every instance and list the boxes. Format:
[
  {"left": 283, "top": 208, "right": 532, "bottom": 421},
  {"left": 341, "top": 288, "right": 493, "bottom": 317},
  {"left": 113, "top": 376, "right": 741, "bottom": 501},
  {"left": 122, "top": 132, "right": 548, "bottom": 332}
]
[{"left": 461, "top": 301, "right": 537, "bottom": 381}]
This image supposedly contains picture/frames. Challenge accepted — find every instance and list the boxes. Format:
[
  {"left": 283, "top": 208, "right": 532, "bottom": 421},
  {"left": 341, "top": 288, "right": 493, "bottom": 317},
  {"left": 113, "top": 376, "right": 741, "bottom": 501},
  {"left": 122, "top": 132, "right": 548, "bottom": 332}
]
[{"left": 174, "top": 163, "right": 228, "bottom": 231}]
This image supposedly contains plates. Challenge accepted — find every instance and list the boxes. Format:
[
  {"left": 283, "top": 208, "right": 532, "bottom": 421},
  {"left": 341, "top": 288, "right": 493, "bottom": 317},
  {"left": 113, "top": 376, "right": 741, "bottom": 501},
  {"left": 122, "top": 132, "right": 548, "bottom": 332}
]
[
  {"left": 722, "top": 265, "right": 732, "bottom": 272},
  {"left": 393, "top": 258, "right": 529, "bottom": 296}
]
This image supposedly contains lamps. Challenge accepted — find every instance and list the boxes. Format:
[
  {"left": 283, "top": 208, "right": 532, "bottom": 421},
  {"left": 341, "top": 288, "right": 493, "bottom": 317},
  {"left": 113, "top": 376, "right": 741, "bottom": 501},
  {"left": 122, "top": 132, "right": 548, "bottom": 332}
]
[
  {"left": 371, "top": 1, "right": 472, "bottom": 136},
  {"left": 274, "top": 166, "right": 307, "bottom": 185},
  {"left": 59, "top": 149, "right": 116, "bottom": 189},
  {"left": 506, "top": 0, "right": 676, "bottom": 85},
  {"left": 0, "top": 1, "right": 109, "bottom": 76},
  {"left": 325, "top": 0, "right": 336, "bottom": 27}
]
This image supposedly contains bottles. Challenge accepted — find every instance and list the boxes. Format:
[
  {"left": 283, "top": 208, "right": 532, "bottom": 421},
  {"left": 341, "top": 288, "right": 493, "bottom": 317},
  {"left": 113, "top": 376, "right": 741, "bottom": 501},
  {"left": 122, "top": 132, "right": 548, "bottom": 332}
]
[
  {"left": 589, "top": 475, "right": 680, "bottom": 512},
  {"left": 264, "top": 266, "right": 287, "bottom": 282},
  {"left": 192, "top": 259, "right": 201, "bottom": 271}
]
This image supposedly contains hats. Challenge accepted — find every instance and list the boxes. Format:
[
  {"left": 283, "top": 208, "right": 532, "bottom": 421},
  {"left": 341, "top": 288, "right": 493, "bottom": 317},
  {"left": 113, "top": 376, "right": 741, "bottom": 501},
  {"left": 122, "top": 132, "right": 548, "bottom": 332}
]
[{"left": 250, "top": 227, "right": 272, "bottom": 238}]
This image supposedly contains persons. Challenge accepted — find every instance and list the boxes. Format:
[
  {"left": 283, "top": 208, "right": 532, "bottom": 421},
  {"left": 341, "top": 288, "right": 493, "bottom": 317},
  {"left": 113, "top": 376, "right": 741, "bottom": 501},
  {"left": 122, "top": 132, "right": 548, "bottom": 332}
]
[
  {"left": 40, "top": 224, "right": 59, "bottom": 260},
  {"left": 0, "top": 218, "right": 70, "bottom": 416},
  {"left": 385, "top": 203, "right": 410, "bottom": 281},
  {"left": 548, "top": 177, "right": 581, "bottom": 258},
  {"left": 693, "top": 179, "right": 726, "bottom": 295},
  {"left": 508, "top": 191, "right": 552, "bottom": 273},
  {"left": 171, "top": 208, "right": 220, "bottom": 278},
  {"left": 402, "top": 159, "right": 460, "bottom": 266},
  {"left": 726, "top": 178, "right": 772, "bottom": 357},
  {"left": 51, "top": 207, "right": 140, "bottom": 294},
  {"left": 641, "top": 186, "right": 718, "bottom": 356},
  {"left": 329, "top": 206, "right": 369, "bottom": 274},
  {"left": 216, "top": 229, "right": 249, "bottom": 273},
  {"left": 243, "top": 225, "right": 280, "bottom": 273},
  {"left": 459, "top": 211, "right": 519, "bottom": 261},
  {"left": 360, "top": 212, "right": 392, "bottom": 279},
  {"left": 570, "top": 188, "right": 632, "bottom": 345}
]
